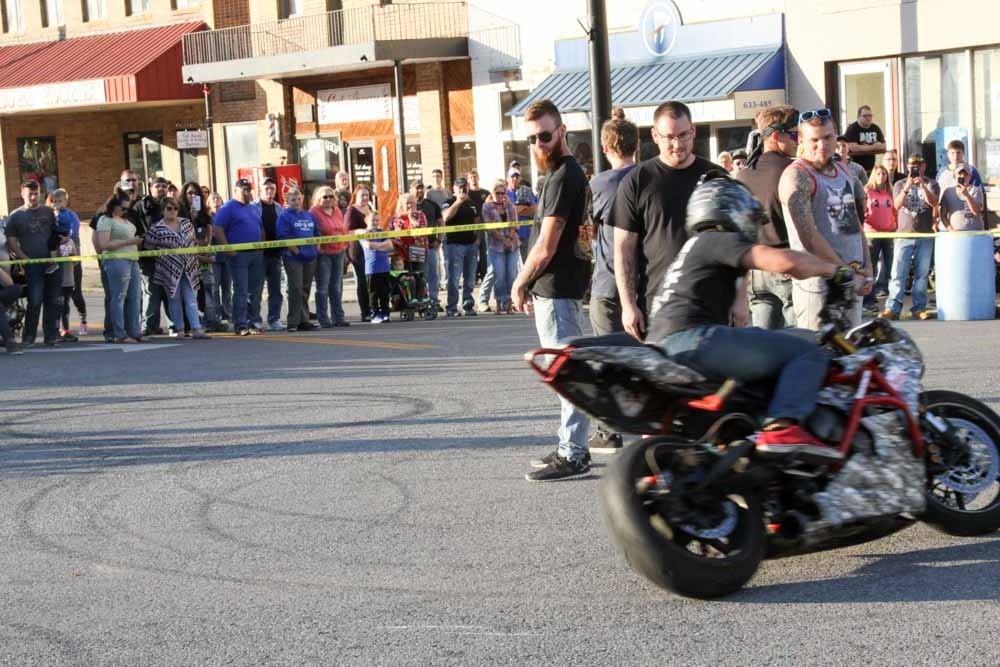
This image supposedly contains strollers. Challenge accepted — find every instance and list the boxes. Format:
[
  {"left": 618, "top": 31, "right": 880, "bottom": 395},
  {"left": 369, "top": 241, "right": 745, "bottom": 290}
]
[{"left": 385, "top": 239, "right": 438, "bottom": 323}]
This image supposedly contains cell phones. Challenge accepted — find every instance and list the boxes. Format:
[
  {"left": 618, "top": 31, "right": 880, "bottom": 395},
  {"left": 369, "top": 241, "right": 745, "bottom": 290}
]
[
  {"left": 192, "top": 196, "right": 201, "bottom": 210},
  {"left": 958, "top": 177, "right": 964, "bottom": 187},
  {"left": 911, "top": 166, "right": 919, "bottom": 177},
  {"left": 463, "top": 187, "right": 467, "bottom": 194}
]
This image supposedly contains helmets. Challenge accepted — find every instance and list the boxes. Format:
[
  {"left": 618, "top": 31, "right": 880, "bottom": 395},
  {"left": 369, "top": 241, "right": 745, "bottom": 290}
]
[{"left": 685, "top": 168, "right": 770, "bottom": 243}]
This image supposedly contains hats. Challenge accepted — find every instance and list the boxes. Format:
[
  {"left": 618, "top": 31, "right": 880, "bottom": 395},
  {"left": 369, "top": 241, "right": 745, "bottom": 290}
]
[
  {"left": 953, "top": 162, "right": 970, "bottom": 177},
  {"left": 410, "top": 179, "right": 424, "bottom": 188},
  {"left": 836, "top": 135, "right": 848, "bottom": 142},
  {"left": 236, "top": 179, "right": 251, "bottom": 186},
  {"left": 454, "top": 176, "right": 467, "bottom": 187},
  {"left": 508, "top": 168, "right": 521, "bottom": 177},
  {"left": 510, "top": 160, "right": 520, "bottom": 167},
  {"left": 114, "top": 181, "right": 134, "bottom": 194},
  {"left": 152, "top": 177, "right": 168, "bottom": 184}
]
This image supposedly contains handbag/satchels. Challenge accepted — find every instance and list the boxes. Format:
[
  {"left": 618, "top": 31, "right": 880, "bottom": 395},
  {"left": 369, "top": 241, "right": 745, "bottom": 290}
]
[{"left": 409, "top": 246, "right": 426, "bottom": 263}]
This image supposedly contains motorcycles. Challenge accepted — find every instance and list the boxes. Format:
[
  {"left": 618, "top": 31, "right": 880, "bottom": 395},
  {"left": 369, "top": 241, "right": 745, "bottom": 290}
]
[{"left": 523, "top": 270, "right": 1000, "bottom": 601}]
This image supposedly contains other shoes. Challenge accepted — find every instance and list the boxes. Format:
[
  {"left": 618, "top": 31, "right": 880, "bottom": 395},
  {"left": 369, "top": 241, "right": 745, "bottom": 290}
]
[
  {"left": 4, "top": 299, "right": 535, "bottom": 355},
  {"left": 861, "top": 288, "right": 932, "bottom": 321}
]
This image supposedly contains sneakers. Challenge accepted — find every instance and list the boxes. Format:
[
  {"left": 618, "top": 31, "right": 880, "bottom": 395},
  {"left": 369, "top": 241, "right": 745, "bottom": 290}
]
[
  {"left": 529, "top": 445, "right": 591, "bottom": 465},
  {"left": 587, "top": 429, "right": 624, "bottom": 454},
  {"left": 525, "top": 456, "right": 592, "bottom": 482},
  {"left": 756, "top": 424, "right": 845, "bottom": 464}
]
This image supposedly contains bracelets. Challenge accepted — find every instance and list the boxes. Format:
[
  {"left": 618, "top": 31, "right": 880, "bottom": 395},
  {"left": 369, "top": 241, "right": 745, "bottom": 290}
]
[
  {"left": 506, "top": 235, "right": 513, "bottom": 240},
  {"left": 899, "top": 186, "right": 910, "bottom": 199},
  {"left": 437, "top": 240, "right": 441, "bottom": 243}
]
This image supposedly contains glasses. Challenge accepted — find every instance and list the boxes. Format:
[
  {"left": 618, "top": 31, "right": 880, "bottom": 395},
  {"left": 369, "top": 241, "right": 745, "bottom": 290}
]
[
  {"left": 164, "top": 206, "right": 178, "bottom": 211},
  {"left": 798, "top": 108, "right": 832, "bottom": 124},
  {"left": 321, "top": 195, "right": 334, "bottom": 199},
  {"left": 125, "top": 179, "right": 137, "bottom": 182},
  {"left": 780, "top": 129, "right": 798, "bottom": 141},
  {"left": 185, "top": 191, "right": 200, "bottom": 195},
  {"left": 494, "top": 191, "right": 505, "bottom": 195},
  {"left": 526, "top": 125, "right": 560, "bottom": 144},
  {"left": 656, "top": 127, "right": 692, "bottom": 142},
  {"left": 119, "top": 204, "right": 130, "bottom": 212}
]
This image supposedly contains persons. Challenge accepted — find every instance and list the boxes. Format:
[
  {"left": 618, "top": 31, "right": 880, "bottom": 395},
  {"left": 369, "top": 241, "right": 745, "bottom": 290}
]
[
  {"left": 0, "top": 178, "right": 88, "bottom": 355},
  {"left": 736, "top": 107, "right": 873, "bottom": 332},
  {"left": 716, "top": 111, "right": 764, "bottom": 178},
  {"left": 88, "top": 160, "right": 546, "bottom": 343},
  {"left": 588, "top": 101, "right": 731, "bottom": 454},
  {"left": 511, "top": 99, "right": 594, "bottom": 483},
  {"left": 642, "top": 178, "right": 860, "bottom": 459},
  {"left": 835, "top": 106, "right": 987, "bottom": 320}
]
